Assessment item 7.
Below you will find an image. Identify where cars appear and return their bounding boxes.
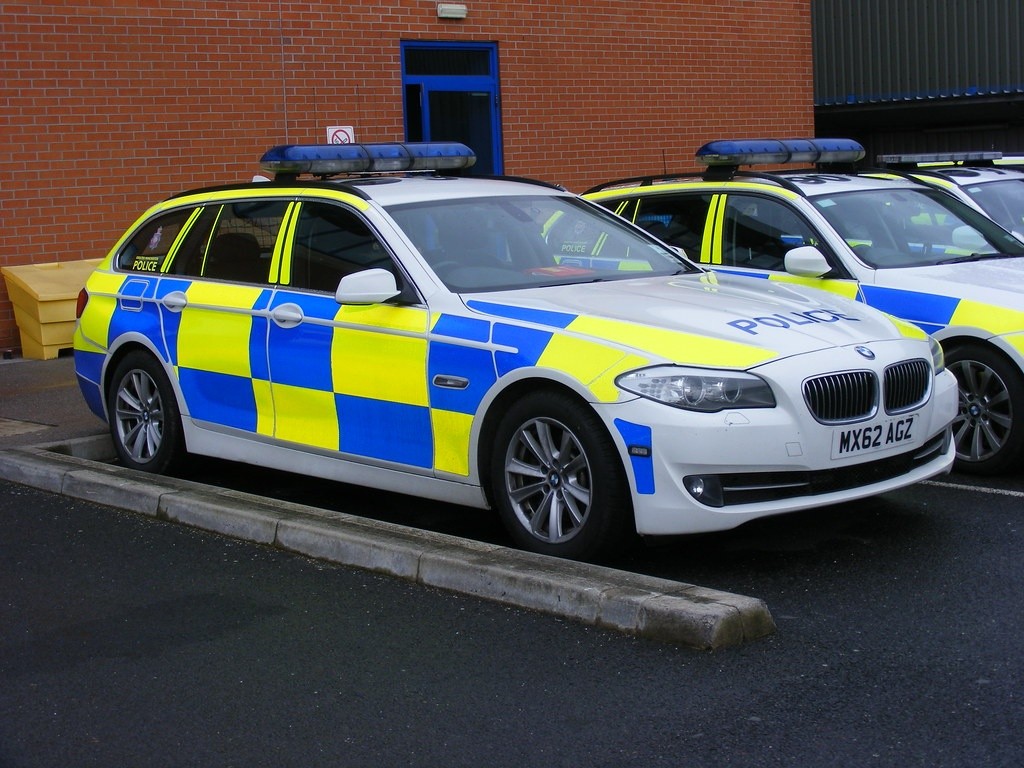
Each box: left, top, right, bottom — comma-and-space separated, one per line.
531, 138, 1024, 479
838, 150, 1024, 260
76, 138, 966, 576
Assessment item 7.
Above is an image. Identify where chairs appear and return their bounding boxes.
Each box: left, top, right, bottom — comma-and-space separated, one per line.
211, 233, 268, 284
